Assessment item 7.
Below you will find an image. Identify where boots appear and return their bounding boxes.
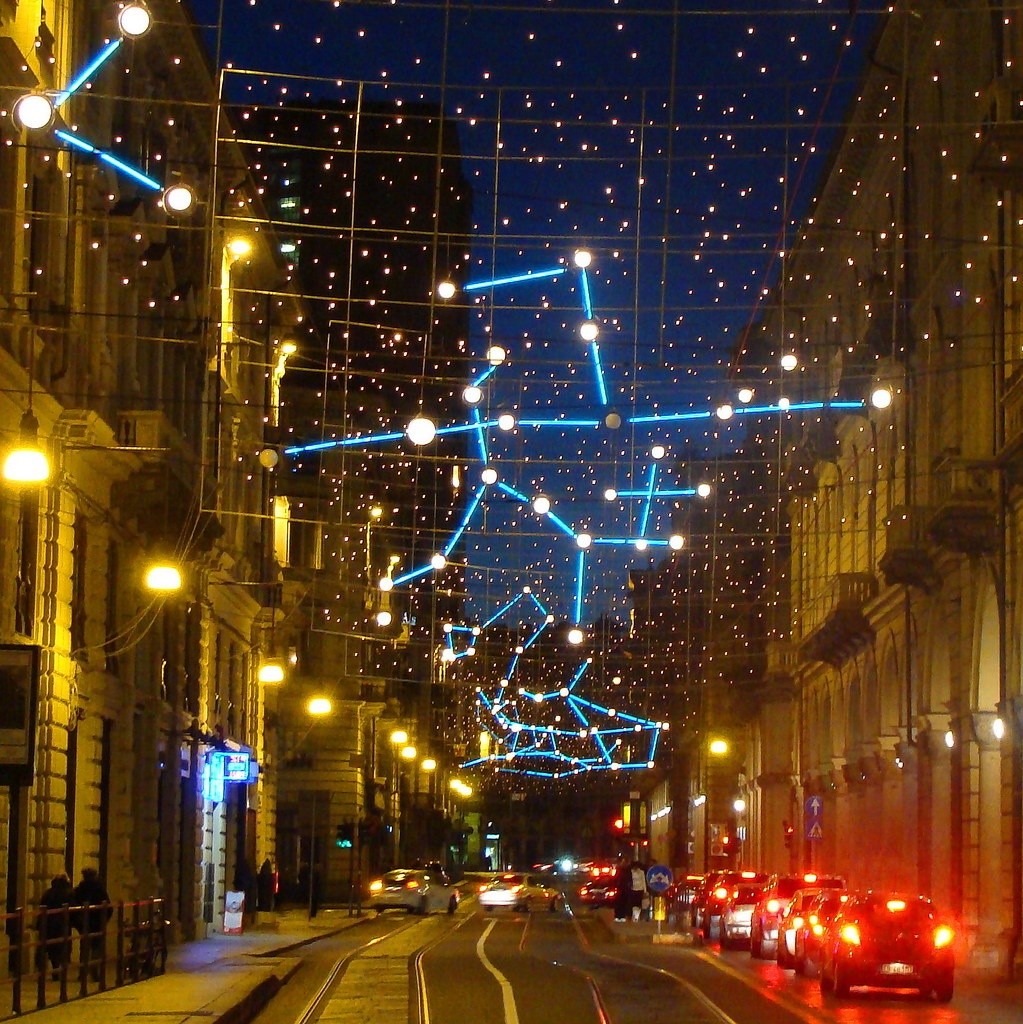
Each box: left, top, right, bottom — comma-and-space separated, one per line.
632, 907, 641, 922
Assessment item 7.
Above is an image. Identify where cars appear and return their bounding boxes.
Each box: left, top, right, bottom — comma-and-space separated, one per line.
369, 868, 460, 915
818, 889, 954, 1004
578, 874, 619, 904
750, 873, 847, 960
795, 890, 852, 978
775, 888, 823, 971
478, 873, 565, 912
669, 868, 768, 938
718, 884, 760, 948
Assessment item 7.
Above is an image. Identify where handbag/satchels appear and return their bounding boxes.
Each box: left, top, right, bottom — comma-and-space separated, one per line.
642, 893, 650, 910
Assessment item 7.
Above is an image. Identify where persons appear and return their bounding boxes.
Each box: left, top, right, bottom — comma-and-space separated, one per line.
631, 862, 647, 923
74, 866, 114, 982
35, 872, 73, 981
613, 860, 634, 922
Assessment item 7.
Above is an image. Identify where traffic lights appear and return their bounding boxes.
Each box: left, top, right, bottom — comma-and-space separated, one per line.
336, 824, 350, 848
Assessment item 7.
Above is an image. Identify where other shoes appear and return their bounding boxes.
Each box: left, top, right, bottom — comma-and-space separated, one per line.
92, 975, 100, 982
52, 973, 59, 980
615, 918, 626, 922
